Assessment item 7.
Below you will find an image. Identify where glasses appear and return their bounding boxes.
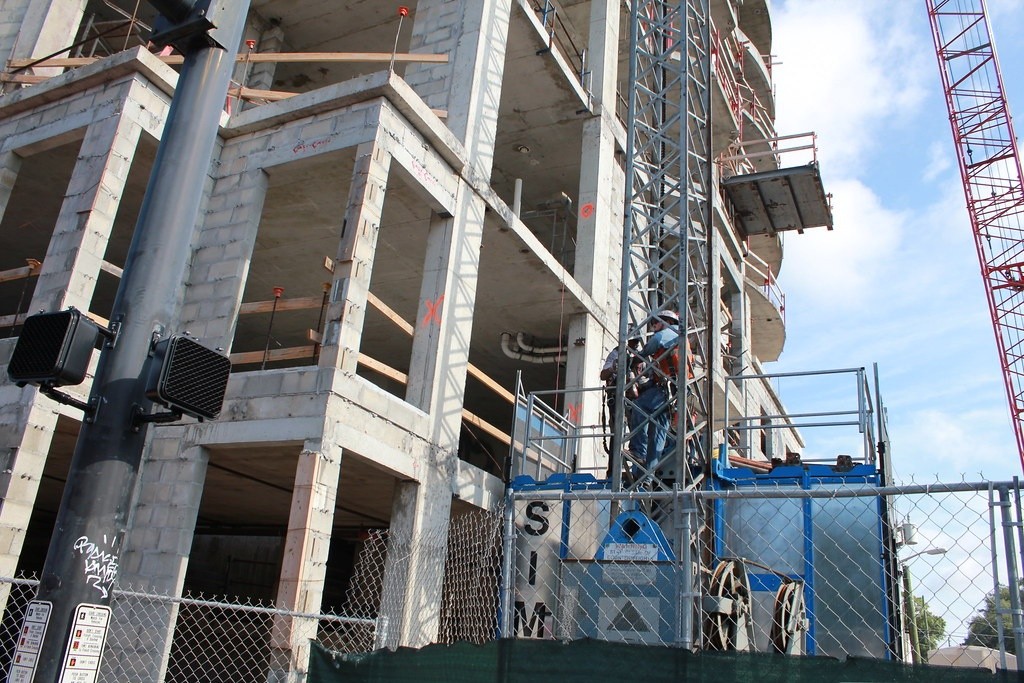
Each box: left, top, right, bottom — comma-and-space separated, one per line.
654, 319, 670, 324
629, 340, 639, 344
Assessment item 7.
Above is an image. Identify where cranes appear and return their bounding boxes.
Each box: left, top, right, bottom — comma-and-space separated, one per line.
503, 1, 1023, 680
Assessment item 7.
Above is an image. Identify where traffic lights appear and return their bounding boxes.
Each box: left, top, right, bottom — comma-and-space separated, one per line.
8, 308, 99, 388
146, 333, 232, 421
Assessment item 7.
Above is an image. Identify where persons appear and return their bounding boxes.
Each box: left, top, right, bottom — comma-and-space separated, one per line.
600, 323, 647, 483
628, 310, 692, 489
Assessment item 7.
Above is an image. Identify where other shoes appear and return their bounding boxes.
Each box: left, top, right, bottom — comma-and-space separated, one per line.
630, 477, 649, 490
624, 450, 645, 464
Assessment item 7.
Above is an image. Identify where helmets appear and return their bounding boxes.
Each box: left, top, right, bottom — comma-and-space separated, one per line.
650, 310, 680, 325
626, 324, 643, 339
